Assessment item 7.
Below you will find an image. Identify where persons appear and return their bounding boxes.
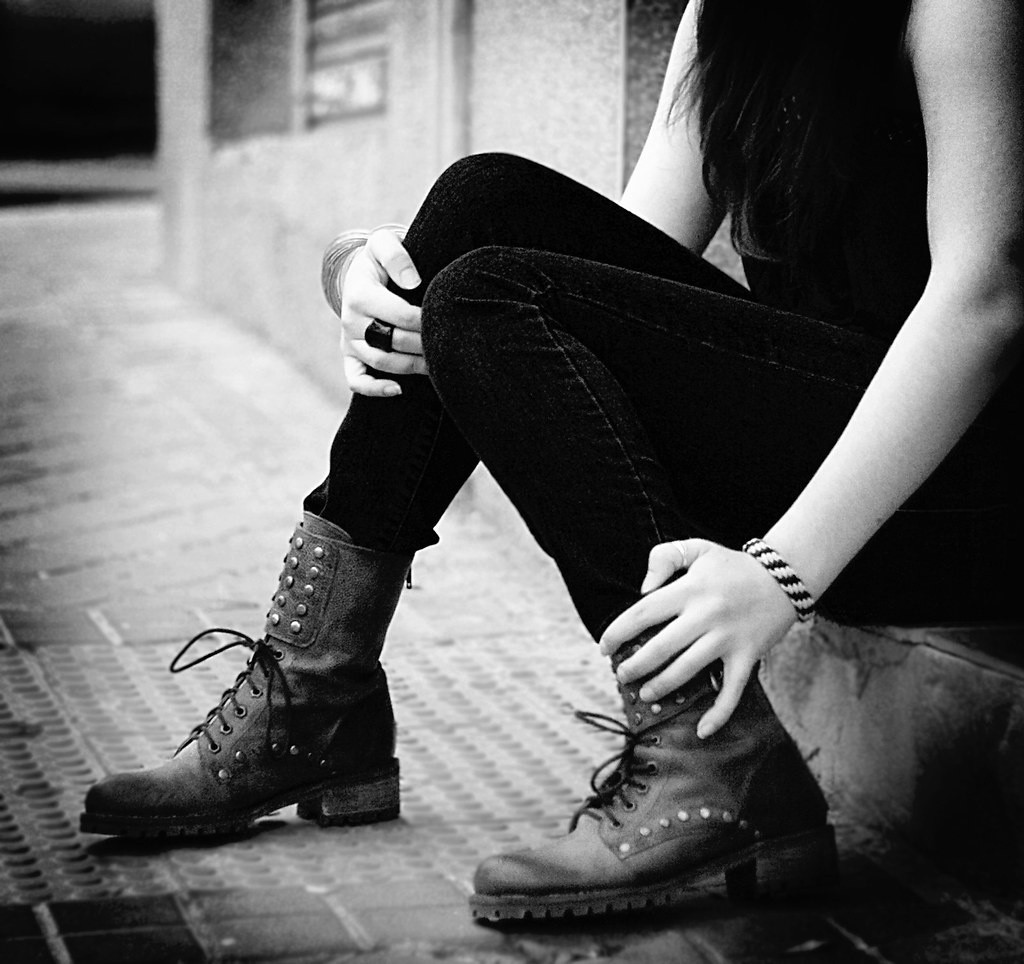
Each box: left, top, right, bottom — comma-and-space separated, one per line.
73, 0, 1023, 928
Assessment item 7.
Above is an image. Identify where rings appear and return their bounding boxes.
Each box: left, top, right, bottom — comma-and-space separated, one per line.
364, 318, 395, 354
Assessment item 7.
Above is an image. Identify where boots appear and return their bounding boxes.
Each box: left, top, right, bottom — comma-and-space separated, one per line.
80, 508, 403, 841
476, 633, 836, 927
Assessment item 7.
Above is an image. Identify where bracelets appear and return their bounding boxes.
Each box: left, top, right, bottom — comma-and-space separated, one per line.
742, 538, 816, 623
